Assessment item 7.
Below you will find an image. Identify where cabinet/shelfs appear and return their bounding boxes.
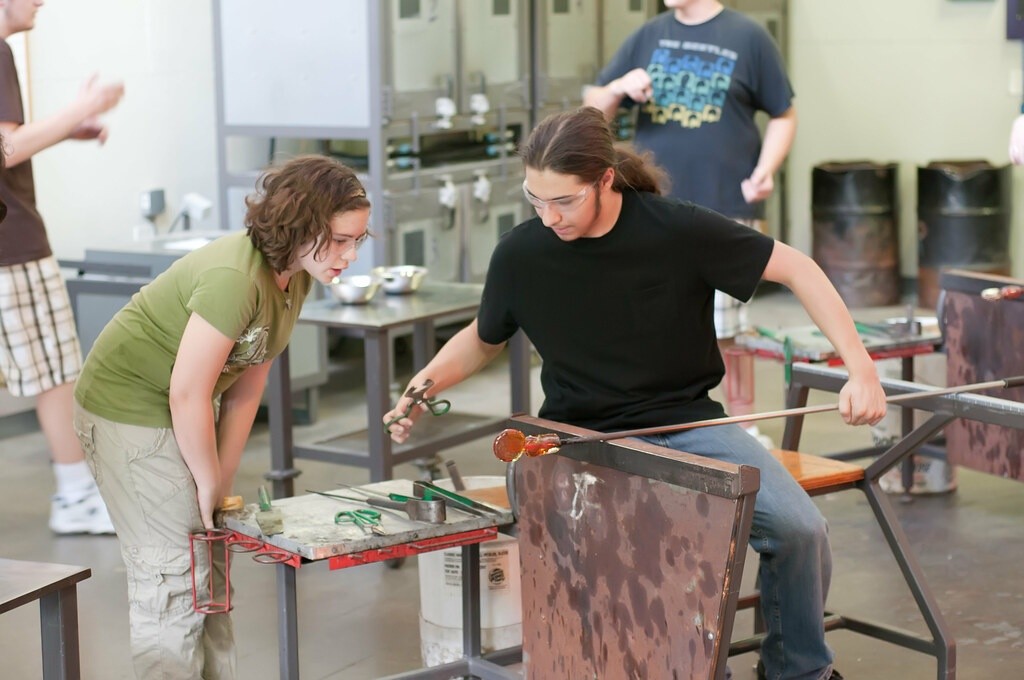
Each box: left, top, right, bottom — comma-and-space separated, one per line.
62, 226, 330, 423
210, 1, 531, 168
532, 1, 661, 128
717, 2, 789, 85
225, 146, 540, 338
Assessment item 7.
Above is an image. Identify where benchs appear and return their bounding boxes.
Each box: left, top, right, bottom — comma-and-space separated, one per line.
211, 360, 1024, 680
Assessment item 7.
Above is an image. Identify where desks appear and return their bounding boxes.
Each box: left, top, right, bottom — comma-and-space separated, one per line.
0, 558, 97, 679
734, 314, 943, 505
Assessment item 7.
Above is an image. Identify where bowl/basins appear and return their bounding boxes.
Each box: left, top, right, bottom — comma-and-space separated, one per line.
376, 264, 425, 293
332, 276, 378, 304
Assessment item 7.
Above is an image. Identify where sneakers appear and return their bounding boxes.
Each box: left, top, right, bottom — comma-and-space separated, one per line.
50, 491, 119, 534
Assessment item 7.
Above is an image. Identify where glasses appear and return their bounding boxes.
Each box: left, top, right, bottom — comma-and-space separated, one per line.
519, 172, 599, 212
319, 235, 368, 255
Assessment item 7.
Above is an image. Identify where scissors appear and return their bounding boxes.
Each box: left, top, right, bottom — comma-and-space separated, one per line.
384, 379, 451, 435
335, 509, 388, 536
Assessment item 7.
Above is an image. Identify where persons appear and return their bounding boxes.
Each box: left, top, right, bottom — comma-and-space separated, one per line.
382, 106, 888, 680
70, 153, 373, 680
0, 0, 124, 536
581, 1, 798, 448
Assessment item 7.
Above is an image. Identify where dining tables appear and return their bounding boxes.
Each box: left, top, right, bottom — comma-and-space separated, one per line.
264, 277, 532, 502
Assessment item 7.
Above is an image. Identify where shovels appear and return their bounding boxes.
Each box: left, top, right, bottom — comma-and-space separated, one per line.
255, 486, 284, 537
